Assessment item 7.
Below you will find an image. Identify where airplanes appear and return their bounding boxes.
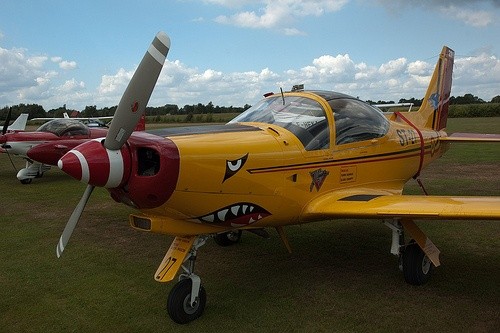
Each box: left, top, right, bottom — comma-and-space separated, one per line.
31, 112, 114, 129
53, 27, 500, 326
264, 90, 414, 134
0, 107, 147, 185
